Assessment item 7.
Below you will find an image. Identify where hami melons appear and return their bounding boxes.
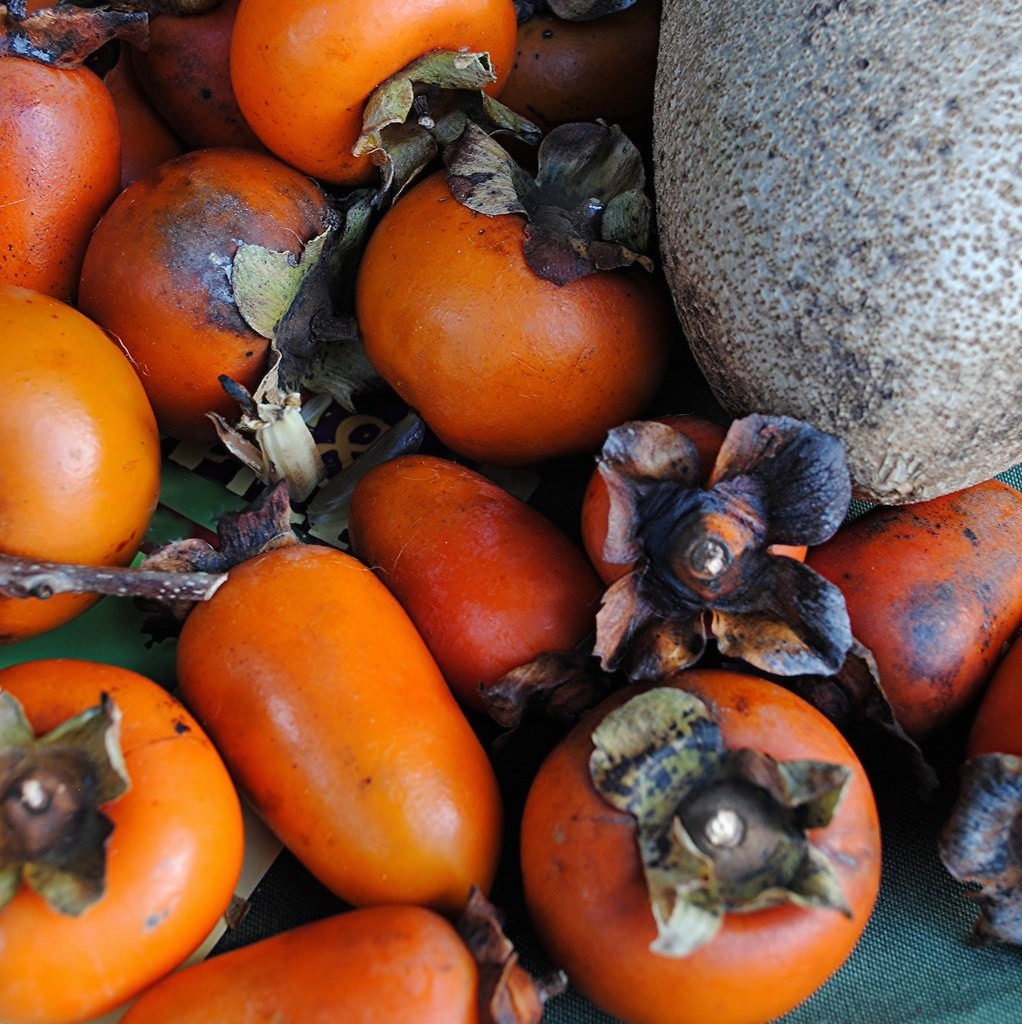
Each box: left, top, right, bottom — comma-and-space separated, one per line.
648, 0, 1022, 517
